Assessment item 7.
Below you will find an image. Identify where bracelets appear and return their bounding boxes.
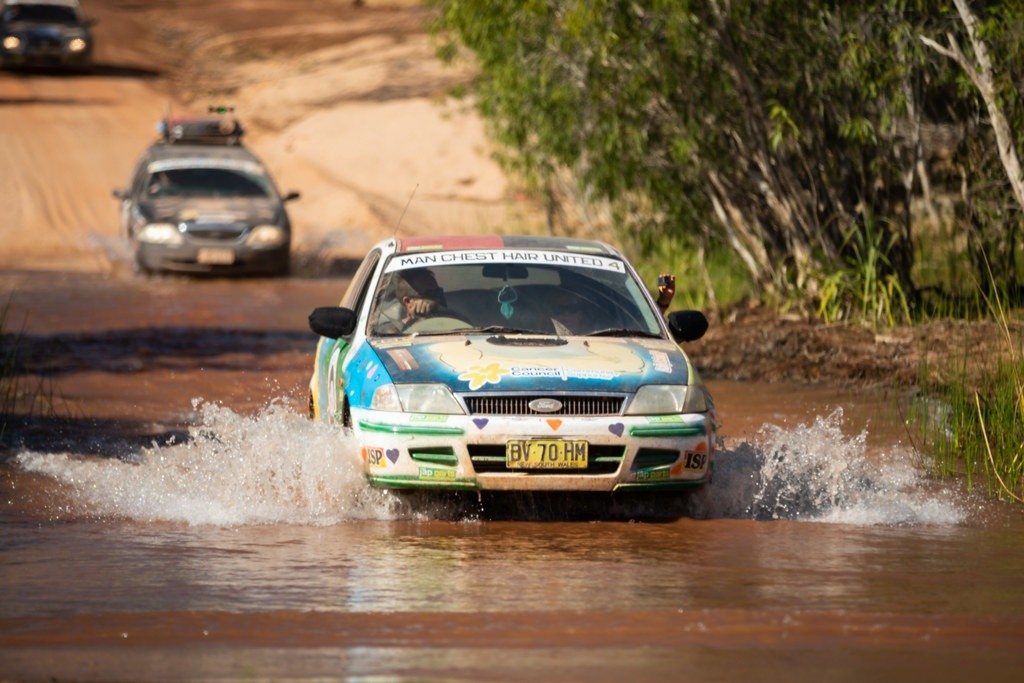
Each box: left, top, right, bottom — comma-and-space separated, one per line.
656, 300, 669, 309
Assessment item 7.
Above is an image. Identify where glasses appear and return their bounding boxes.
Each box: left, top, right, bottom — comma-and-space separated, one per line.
418, 287, 443, 298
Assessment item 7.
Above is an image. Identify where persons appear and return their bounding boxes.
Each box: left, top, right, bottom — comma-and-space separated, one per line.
375, 267, 460, 337
149, 173, 180, 197
539, 273, 675, 337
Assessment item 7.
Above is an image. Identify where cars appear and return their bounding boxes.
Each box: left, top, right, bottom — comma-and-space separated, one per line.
0, 0, 97, 77
110, 116, 301, 281
309, 234, 719, 504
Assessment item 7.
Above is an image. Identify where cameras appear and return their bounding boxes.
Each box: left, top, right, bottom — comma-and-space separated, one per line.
657, 277, 671, 287
